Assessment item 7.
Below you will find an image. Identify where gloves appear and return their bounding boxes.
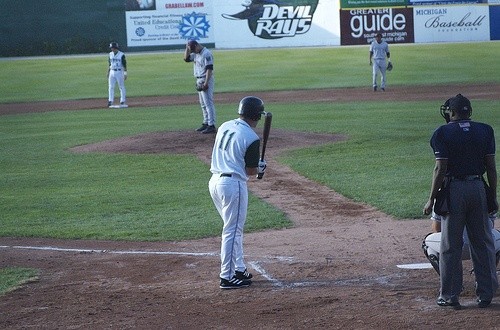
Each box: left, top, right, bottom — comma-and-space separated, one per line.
256, 158, 267, 174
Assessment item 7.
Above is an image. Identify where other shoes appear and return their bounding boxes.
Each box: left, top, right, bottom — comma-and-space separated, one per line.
437, 300, 460, 307
476, 296, 491, 307
195, 124, 216, 134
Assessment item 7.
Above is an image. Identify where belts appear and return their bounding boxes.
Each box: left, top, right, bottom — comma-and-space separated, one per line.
113, 69, 121, 70
452, 175, 480, 181
197, 77, 203, 78
220, 174, 232, 177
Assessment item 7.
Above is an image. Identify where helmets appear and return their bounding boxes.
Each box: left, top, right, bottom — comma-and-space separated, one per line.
109, 42, 119, 49
238, 95, 267, 115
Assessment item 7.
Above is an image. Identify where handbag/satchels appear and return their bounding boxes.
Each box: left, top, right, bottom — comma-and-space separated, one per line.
434, 173, 450, 216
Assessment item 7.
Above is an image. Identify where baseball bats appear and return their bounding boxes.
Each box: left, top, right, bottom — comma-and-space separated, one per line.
255, 112, 272, 180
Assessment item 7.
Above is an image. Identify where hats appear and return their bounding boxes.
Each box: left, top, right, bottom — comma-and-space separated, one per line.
375, 33, 382, 37
188, 41, 198, 53
445, 97, 471, 113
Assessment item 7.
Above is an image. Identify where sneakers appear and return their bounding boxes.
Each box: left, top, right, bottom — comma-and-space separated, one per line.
219, 275, 252, 288
234, 267, 253, 279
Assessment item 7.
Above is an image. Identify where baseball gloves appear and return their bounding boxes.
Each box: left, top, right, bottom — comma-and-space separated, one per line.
196, 80, 209, 92
386, 61, 394, 72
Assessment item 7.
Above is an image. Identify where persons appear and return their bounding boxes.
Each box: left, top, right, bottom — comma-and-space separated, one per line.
183, 40, 217, 134
208, 95, 268, 289
419, 93, 500, 308
369, 34, 391, 93
106, 42, 128, 107
